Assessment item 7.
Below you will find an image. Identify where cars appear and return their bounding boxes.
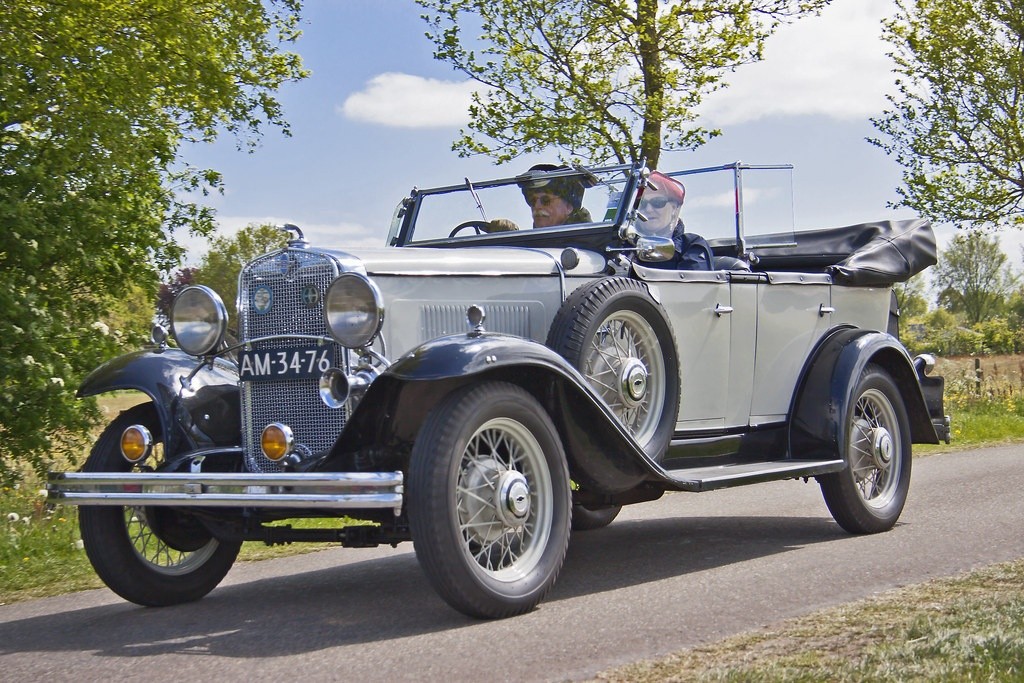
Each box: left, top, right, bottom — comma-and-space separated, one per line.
45, 160, 954, 621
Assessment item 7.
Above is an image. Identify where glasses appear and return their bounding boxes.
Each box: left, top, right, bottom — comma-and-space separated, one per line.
639, 196, 675, 209
530, 194, 560, 207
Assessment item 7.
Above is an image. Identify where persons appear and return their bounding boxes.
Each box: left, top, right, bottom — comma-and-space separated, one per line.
485, 164, 592, 233
634, 170, 715, 270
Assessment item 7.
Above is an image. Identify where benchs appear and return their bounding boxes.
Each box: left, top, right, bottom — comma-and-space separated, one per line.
713, 256, 752, 272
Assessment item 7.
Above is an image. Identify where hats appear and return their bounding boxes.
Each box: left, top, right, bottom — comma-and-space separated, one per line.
519, 164, 585, 210
643, 171, 685, 205
